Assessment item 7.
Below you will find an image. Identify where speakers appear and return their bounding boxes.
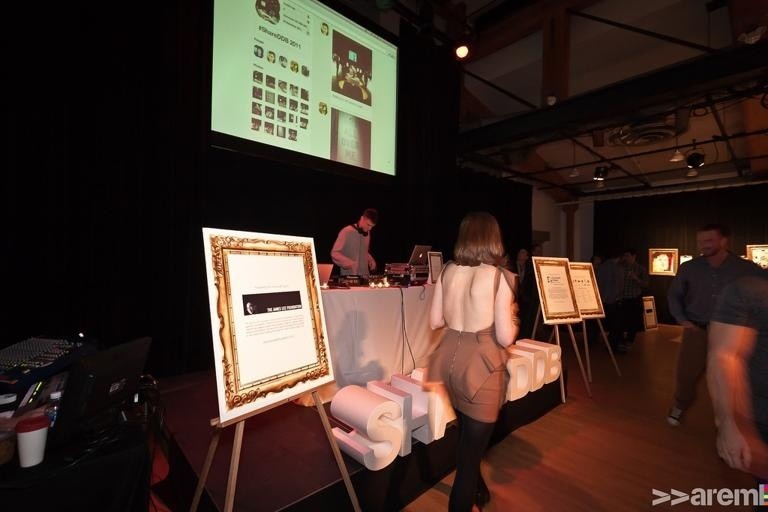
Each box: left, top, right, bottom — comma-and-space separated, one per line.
0, 423, 153, 511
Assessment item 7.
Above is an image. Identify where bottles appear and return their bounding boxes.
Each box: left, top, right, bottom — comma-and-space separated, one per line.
45, 392, 62, 427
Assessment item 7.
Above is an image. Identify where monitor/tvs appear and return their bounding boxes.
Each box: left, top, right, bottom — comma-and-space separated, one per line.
203, 0, 407, 190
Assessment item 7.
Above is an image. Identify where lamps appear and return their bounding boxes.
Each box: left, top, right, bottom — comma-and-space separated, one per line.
593, 165, 620, 182
669, 135, 685, 162
684, 138, 706, 169
568, 142, 579, 177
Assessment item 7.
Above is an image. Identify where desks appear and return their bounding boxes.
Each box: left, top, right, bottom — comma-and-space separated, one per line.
292, 283, 435, 407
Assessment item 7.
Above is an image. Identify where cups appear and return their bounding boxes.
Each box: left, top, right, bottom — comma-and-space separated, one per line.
15, 416, 51, 467
0, 393, 17, 419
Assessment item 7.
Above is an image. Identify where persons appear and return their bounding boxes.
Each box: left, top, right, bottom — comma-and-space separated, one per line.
425, 211, 519, 511
331, 206, 379, 276
704, 268, 768, 512
587, 248, 651, 354
512, 242, 547, 342
666, 220, 760, 428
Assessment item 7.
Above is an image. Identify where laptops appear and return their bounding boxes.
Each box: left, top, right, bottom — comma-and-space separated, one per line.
392, 245, 432, 265
317, 263, 334, 285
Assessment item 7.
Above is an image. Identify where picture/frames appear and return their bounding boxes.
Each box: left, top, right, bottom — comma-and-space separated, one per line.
648, 248, 678, 276
746, 245, 768, 269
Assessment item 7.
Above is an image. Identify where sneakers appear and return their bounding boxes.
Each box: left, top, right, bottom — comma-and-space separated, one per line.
666, 405, 682, 426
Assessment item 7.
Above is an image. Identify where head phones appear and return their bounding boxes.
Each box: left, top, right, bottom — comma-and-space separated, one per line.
356, 222, 368, 237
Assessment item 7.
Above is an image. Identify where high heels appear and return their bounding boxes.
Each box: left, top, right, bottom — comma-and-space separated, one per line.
474, 491, 489, 511
